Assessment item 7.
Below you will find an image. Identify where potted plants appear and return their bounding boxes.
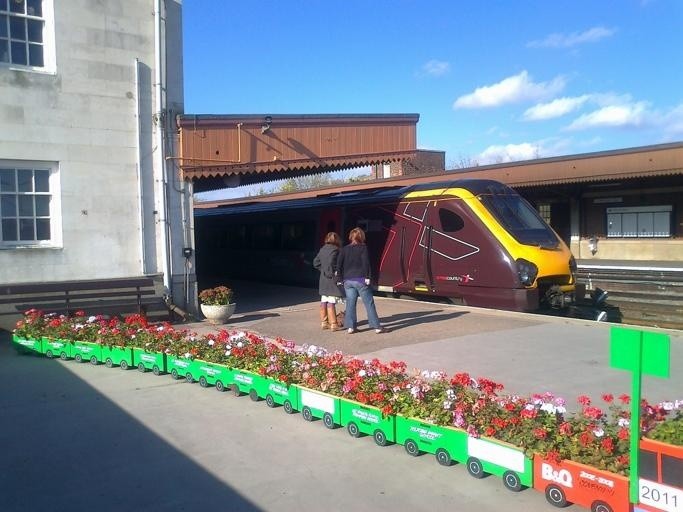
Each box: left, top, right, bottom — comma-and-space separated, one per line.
199, 286, 236, 326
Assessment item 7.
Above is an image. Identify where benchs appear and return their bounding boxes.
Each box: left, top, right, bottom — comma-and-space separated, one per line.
7, 279, 174, 323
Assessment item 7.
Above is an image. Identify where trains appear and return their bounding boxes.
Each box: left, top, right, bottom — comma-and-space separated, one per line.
191, 178, 577, 314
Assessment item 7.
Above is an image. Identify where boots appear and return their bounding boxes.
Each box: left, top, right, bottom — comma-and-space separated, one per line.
318, 305, 330, 330
325, 306, 341, 332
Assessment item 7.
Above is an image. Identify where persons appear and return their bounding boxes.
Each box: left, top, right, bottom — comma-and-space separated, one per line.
312, 232, 344, 332
334, 226, 384, 334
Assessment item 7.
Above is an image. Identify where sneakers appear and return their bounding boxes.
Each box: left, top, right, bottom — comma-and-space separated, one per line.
347, 326, 392, 334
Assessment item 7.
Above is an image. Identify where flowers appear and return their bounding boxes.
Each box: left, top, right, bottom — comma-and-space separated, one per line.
265, 337, 408, 417
407, 368, 682, 475
13, 307, 125, 346
124, 314, 267, 377
